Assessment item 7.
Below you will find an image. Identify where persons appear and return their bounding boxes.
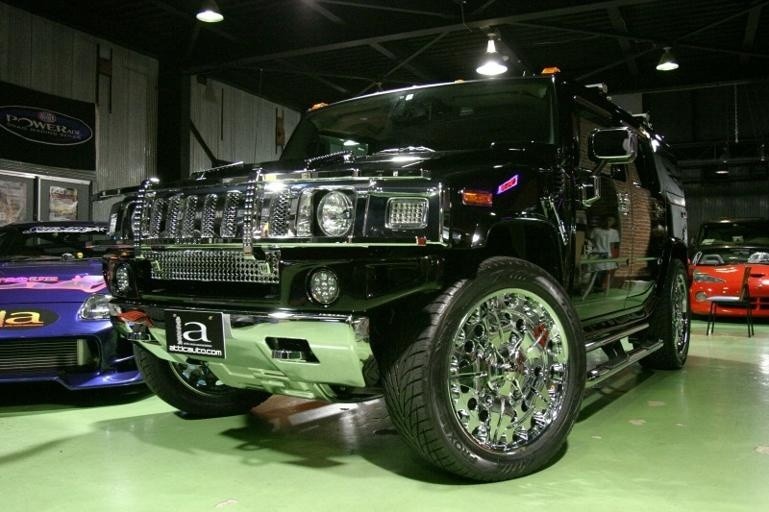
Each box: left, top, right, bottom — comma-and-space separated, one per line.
588, 214, 621, 294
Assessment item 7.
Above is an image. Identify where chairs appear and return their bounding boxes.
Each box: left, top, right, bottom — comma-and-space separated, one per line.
699, 250, 769, 266
705, 265, 754, 337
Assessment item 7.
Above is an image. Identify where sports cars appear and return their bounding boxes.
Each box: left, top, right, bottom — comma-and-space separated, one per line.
0, 218, 152, 402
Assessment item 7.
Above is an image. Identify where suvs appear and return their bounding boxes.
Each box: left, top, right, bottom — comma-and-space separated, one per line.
93, 62, 700, 487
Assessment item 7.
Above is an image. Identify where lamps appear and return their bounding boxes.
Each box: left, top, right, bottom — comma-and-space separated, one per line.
473, 25, 509, 77
194, 0, 224, 23
655, 43, 680, 72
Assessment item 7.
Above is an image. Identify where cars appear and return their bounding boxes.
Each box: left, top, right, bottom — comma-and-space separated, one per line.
685, 216, 769, 323
